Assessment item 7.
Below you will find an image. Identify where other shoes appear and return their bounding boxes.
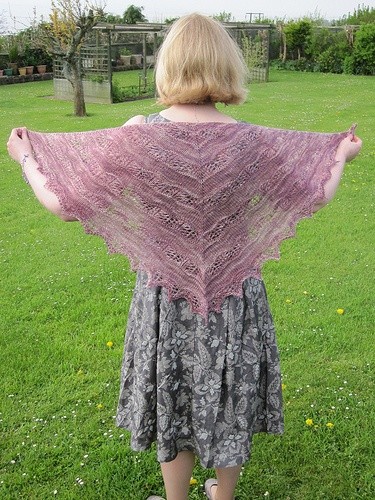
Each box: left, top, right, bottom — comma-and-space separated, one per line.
145, 495, 168, 500
205, 478, 235, 500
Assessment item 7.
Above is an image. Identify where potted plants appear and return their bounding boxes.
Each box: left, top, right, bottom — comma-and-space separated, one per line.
0, 62, 5, 76
4, 62, 13, 75
18, 59, 27, 76
110, 52, 117, 66
7, 43, 19, 75
131, 42, 142, 64
119, 47, 132, 66
36, 48, 48, 74
23, 48, 35, 75
146, 46, 155, 64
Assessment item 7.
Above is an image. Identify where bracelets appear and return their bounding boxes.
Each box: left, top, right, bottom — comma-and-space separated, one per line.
21, 153, 35, 185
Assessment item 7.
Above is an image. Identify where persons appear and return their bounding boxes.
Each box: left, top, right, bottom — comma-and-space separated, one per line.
6, 12, 362, 499
